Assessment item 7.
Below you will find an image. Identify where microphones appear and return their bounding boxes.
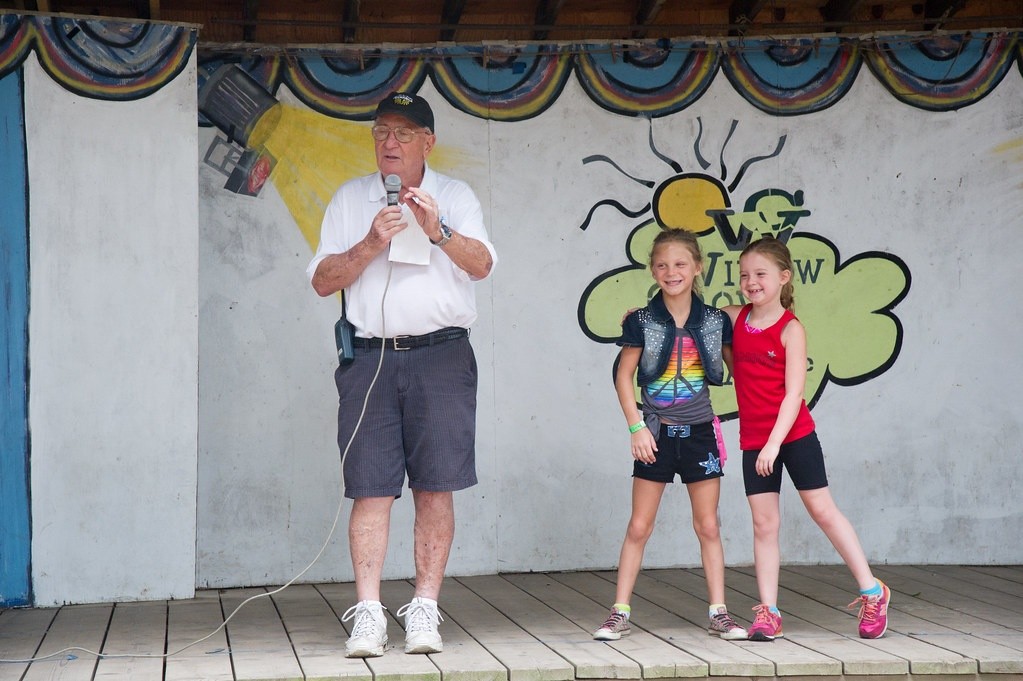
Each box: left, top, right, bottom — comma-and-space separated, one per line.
385, 174, 402, 207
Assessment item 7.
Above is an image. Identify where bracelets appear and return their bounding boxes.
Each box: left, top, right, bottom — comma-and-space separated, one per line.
629, 421, 646, 433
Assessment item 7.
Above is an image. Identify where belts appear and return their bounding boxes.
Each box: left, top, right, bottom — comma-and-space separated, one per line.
352, 328, 468, 350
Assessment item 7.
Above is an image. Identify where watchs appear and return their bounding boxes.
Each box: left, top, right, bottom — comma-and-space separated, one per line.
429, 224, 451, 247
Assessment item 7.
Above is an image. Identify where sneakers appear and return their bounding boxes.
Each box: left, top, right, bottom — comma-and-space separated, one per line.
708, 606, 748, 640
593, 607, 632, 640
341, 600, 390, 658
748, 604, 785, 641
848, 577, 891, 638
396, 597, 444, 653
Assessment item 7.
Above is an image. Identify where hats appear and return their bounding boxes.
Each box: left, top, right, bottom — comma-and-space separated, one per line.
374, 91, 434, 134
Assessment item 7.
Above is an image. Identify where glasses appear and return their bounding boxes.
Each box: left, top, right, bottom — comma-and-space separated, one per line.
372, 124, 428, 143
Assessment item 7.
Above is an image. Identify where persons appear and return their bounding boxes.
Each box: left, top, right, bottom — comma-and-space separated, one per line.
621, 237, 890, 641
592, 229, 747, 640
307, 91, 498, 658
